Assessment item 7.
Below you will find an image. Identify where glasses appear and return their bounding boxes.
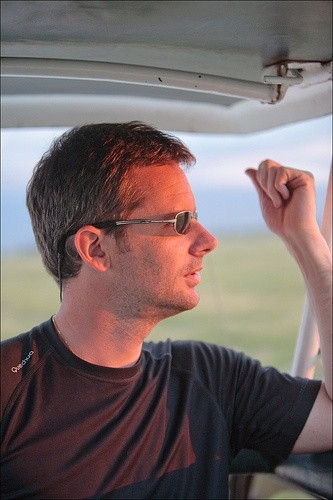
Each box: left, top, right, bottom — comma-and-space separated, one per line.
57, 207, 197, 253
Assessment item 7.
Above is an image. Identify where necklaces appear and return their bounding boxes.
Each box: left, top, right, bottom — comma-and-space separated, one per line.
52, 315, 75, 355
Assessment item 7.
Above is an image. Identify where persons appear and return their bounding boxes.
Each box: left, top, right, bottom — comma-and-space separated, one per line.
1, 120, 332, 499
